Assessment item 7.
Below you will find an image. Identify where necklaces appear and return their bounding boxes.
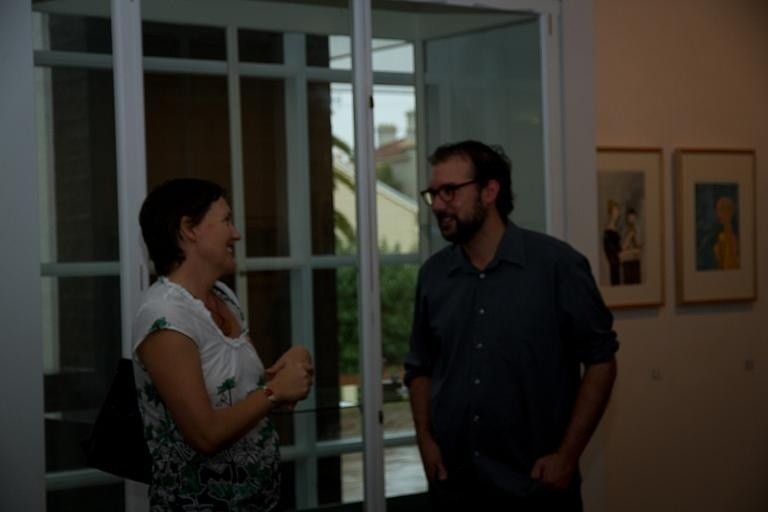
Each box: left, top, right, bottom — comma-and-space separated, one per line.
195, 294, 232, 336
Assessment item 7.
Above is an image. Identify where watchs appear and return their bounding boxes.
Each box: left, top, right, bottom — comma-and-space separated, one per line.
262, 386, 278, 409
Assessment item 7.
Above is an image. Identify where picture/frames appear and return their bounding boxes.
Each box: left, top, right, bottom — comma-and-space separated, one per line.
592, 145, 665, 310
674, 148, 758, 306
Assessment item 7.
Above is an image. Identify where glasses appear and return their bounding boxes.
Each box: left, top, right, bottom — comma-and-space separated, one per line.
421, 179, 484, 201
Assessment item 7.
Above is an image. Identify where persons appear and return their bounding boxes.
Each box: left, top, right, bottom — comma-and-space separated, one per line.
602, 199, 624, 286
132, 179, 314, 510
405, 141, 621, 510
621, 210, 641, 286
712, 195, 739, 268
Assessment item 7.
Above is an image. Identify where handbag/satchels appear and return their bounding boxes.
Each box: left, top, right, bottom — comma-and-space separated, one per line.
83, 358, 153, 486
